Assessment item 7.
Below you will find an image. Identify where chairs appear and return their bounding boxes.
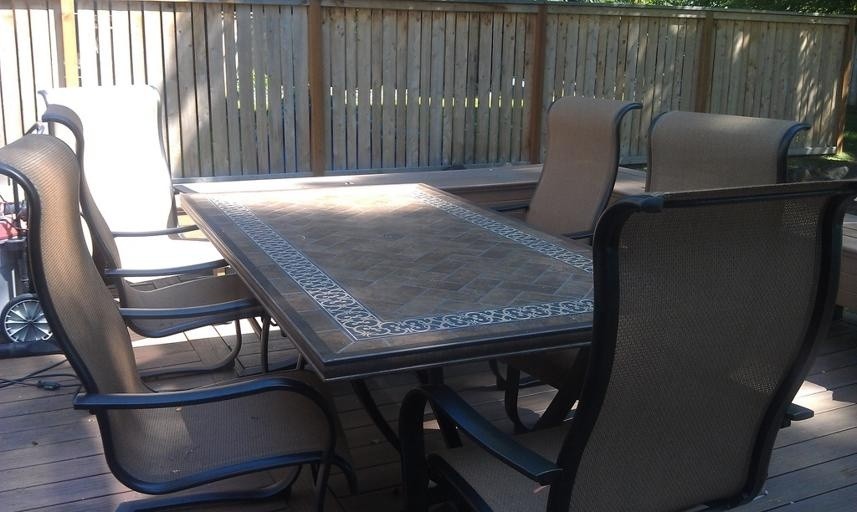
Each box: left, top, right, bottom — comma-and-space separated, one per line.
1, 132, 366, 512
41, 103, 306, 380
398, 177, 857, 511
433, 92, 644, 390
505, 109, 812, 436
39, 84, 234, 290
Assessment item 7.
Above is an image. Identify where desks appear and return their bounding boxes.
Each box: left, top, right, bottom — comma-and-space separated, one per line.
174, 174, 596, 512
